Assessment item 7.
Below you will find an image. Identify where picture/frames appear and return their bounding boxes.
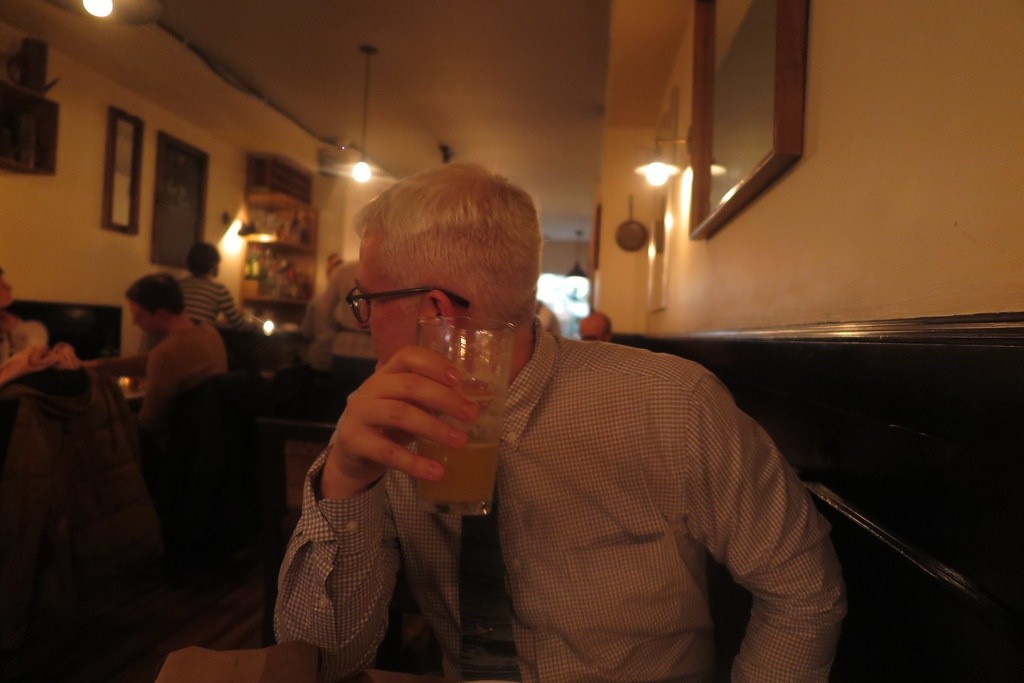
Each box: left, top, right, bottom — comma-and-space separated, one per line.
100, 106, 144, 236
689, 0, 807, 242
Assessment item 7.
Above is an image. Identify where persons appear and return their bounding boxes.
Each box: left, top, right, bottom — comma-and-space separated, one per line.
271, 160, 845, 683
123, 271, 230, 435
0, 270, 50, 400
178, 242, 253, 333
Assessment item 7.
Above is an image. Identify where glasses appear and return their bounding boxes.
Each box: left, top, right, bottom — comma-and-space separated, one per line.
346, 286, 471, 323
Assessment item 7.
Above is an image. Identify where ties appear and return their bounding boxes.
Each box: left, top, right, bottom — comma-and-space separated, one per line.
458, 480, 522, 682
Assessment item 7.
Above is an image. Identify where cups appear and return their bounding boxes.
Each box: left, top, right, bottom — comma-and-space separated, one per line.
416, 315, 516, 515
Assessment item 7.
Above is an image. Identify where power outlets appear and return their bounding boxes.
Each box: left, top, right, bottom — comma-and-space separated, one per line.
238, 189, 318, 306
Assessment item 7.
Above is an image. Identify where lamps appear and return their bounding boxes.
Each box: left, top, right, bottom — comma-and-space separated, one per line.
222, 211, 275, 242
353, 46, 377, 182
566, 232, 589, 282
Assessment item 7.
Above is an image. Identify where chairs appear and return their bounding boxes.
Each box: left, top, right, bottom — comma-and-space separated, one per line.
0, 359, 323, 653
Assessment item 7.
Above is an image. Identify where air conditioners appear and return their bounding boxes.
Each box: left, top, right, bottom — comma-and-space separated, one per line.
635, 137, 686, 186
